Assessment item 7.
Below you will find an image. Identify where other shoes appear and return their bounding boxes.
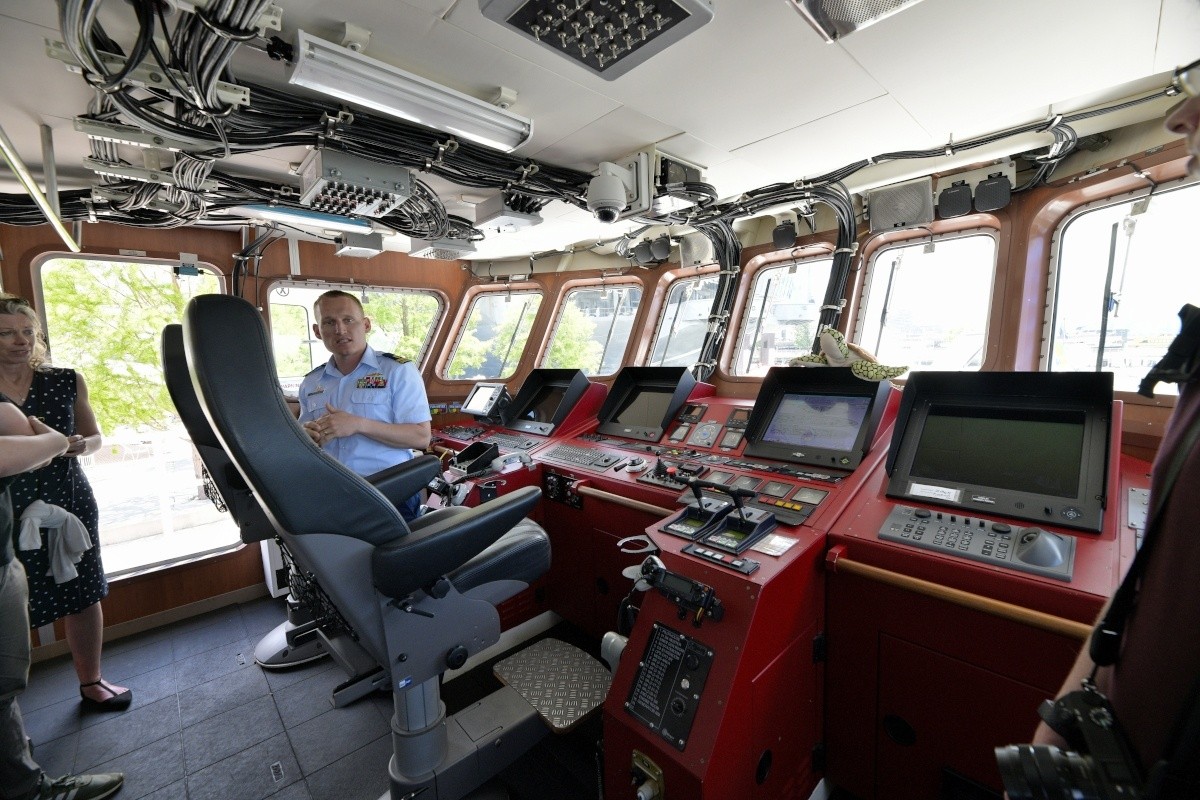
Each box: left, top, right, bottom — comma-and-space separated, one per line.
79, 676, 134, 708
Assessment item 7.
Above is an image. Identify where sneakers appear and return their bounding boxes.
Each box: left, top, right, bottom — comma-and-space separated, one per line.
32, 771, 125, 800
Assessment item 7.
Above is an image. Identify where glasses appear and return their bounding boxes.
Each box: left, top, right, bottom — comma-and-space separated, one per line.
1175, 59, 1200, 98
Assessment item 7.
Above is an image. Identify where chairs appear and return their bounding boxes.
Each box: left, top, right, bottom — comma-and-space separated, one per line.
161, 295, 559, 800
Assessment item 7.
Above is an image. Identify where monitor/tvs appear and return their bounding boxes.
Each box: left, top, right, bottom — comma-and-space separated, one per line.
761, 392, 872, 451
909, 404, 1085, 500
610, 385, 675, 429
460, 382, 506, 416
519, 386, 568, 424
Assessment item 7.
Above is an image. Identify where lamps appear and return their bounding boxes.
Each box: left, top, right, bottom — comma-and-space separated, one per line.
281, 31, 534, 153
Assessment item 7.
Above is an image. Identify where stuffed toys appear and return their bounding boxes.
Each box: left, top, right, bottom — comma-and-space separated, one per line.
789, 328, 911, 382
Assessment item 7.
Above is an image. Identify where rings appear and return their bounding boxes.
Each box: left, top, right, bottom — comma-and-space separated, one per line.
323, 430, 327, 436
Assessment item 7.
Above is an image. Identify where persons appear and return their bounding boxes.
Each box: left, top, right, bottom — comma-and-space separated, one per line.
0, 293, 132, 710
0, 402, 124, 800
296, 290, 432, 523
1003, 303, 1200, 800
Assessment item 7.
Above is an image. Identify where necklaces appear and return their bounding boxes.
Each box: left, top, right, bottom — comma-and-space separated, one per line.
0, 371, 30, 402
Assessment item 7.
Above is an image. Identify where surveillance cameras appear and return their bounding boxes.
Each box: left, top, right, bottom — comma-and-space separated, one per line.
587, 176, 627, 224
